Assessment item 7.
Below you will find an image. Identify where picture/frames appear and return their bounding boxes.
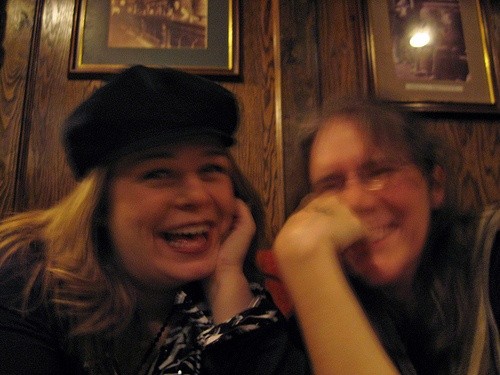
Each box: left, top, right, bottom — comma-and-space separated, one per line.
67, 0, 244, 82
360, 0, 500, 117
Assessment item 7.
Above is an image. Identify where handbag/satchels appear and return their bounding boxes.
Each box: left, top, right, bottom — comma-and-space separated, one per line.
458, 205, 500, 375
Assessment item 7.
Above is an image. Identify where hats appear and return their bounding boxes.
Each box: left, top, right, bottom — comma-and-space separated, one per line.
62, 65, 241, 181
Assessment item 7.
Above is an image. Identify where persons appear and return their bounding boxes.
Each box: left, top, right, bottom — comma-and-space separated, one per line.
272, 101, 500, 375
131, 1, 189, 47
0, 64, 306, 375
394, 0, 460, 78
